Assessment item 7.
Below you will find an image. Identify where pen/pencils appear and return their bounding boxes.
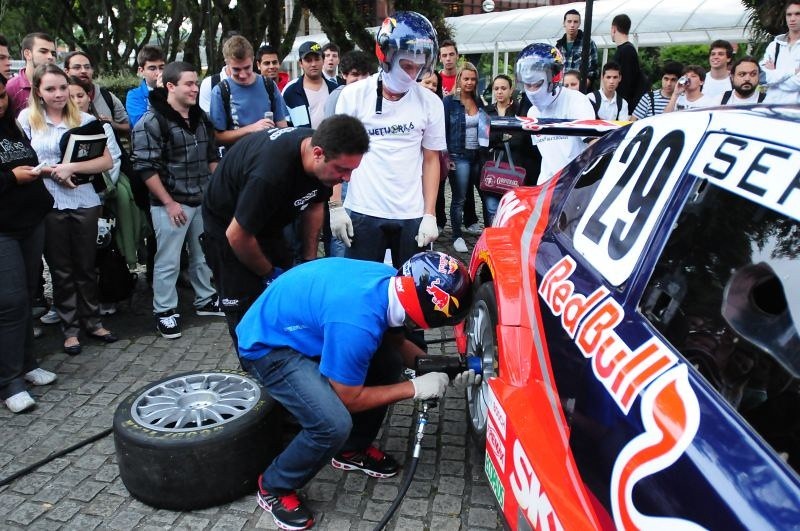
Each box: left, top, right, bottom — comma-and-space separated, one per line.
32, 161, 47, 171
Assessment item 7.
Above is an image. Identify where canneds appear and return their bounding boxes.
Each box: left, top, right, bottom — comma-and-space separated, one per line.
679, 77, 690, 85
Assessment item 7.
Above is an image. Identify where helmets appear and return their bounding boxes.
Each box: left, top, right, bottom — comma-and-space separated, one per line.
395, 248, 472, 330
516, 42, 565, 93
375, 10, 439, 73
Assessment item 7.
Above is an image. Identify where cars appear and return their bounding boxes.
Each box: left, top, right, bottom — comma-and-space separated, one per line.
457, 103, 799, 530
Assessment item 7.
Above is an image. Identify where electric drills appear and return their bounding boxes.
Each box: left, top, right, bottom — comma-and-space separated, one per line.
416, 354, 481, 406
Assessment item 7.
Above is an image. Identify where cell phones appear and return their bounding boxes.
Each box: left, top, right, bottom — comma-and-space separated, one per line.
264, 111, 274, 121
33, 159, 48, 171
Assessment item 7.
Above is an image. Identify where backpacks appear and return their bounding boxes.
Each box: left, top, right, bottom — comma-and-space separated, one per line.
96, 231, 137, 306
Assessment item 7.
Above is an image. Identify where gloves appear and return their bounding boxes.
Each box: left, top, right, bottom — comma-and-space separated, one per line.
415, 215, 439, 250
453, 369, 483, 390
410, 371, 450, 402
330, 206, 354, 248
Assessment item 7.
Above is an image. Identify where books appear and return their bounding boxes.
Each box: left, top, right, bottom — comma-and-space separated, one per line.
63, 134, 108, 185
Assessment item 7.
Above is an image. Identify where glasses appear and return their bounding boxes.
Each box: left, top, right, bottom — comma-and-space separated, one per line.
68, 65, 92, 71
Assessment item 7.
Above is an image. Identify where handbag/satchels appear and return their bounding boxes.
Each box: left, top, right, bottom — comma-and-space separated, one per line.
478, 139, 527, 195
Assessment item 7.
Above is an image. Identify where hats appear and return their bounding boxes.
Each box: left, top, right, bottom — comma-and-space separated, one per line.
300, 41, 322, 59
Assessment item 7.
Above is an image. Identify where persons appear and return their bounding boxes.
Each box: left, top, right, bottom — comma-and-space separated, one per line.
701, 40, 734, 101
199, 114, 369, 430
235, 250, 481, 531
586, 63, 628, 121
713, 57, 772, 105
556, 9, 599, 94
611, 14, 640, 107
665, 313, 800, 474
664, 65, 712, 112
564, 70, 582, 91
759, 0, 800, 103
329, 11, 446, 381
0, 32, 226, 412
419, 41, 542, 252
630, 64, 682, 121
515, 43, 598, 186
198, 31, 372, 264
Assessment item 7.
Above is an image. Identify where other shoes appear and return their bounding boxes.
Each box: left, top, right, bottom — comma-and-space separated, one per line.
40, 310, 62, 323
32, 306, 46, 317
98, 300, 116, 316
87, 326, 117, 342
467, 224, 485, 235
63, 338, 82, 354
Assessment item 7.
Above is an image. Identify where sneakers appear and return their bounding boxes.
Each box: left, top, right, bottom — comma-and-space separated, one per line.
331, 446, 399, 479
155, 308, 183, 340
256, 474, 315, 530
195, 295, 227, 317
5, 391, 36, 413
24, 366, 57, 386
454, 238, 468, 252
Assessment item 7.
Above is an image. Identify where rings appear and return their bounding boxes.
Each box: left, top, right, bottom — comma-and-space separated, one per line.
176, 218, 178, 220
